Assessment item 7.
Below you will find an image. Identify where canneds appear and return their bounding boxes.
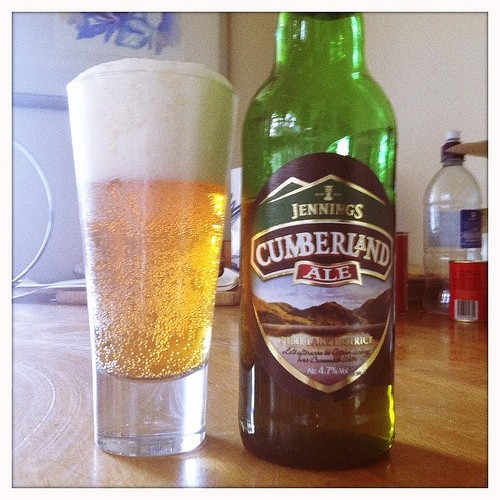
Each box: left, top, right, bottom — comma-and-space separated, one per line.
448, 259, 487, 323
394, 231, 410, 315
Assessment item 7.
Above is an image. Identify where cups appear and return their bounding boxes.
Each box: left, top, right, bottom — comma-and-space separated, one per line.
67, 71, 240, 457
448, 260, 488, 323
395, 232, 408, 320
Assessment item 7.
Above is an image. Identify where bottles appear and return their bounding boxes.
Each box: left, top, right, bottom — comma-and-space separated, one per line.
237, 12, 398, 471
423, 129, 483, 315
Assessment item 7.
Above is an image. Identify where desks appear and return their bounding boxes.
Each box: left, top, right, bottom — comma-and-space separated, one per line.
13, 280, 488, 487
